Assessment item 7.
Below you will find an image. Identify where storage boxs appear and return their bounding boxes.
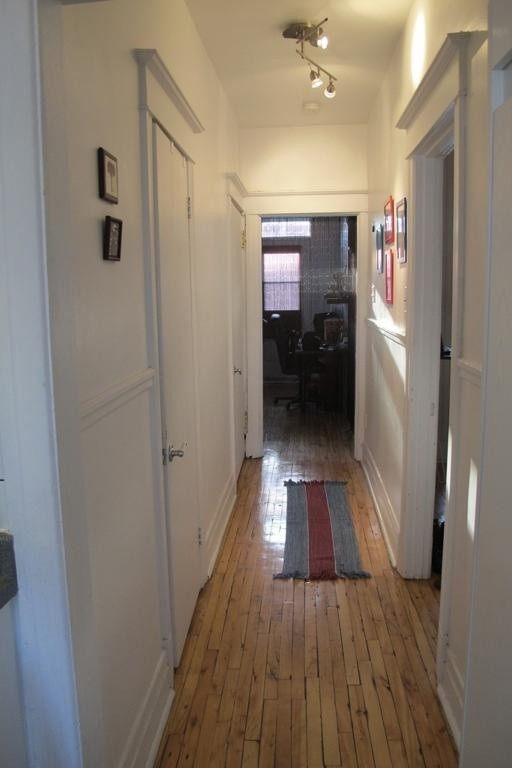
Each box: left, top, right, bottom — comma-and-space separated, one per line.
320, 317, 344, 346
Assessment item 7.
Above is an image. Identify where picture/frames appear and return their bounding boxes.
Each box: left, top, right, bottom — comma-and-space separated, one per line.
372, 193, 410, 311
102, 214, 127, 264
95, 143, 123, 206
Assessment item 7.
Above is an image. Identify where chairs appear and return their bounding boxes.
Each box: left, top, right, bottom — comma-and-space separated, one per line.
271, 308, 323, 414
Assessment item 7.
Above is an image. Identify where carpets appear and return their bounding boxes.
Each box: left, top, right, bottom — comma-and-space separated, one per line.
272, 476, 374, 585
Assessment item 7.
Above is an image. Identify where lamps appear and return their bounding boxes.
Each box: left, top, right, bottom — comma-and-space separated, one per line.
281, 14, 346, 102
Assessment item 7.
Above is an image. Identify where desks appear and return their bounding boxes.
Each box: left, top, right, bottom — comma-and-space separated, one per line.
297, 347, 353, 418
